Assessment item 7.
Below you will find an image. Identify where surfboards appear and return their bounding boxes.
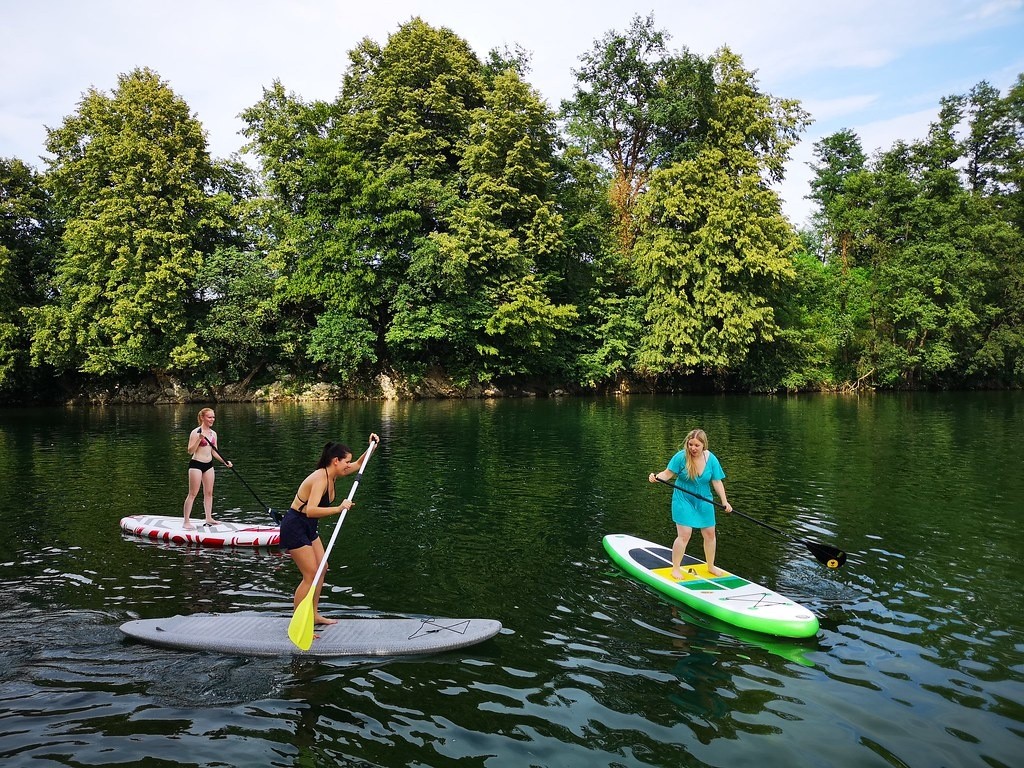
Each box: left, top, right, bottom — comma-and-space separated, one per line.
601, 531, 821, 639
119, 514, 280, 547
118, 616, 503, 653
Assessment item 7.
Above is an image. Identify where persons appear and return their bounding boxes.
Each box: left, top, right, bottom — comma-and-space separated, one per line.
183, 408, 233, 530
649, 429, 733, 580
280, 433, 380, 640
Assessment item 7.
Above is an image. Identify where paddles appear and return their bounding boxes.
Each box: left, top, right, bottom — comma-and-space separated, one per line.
286, 435, 380, 654
197, 427, 285, 529
652, 473, 849, 570
